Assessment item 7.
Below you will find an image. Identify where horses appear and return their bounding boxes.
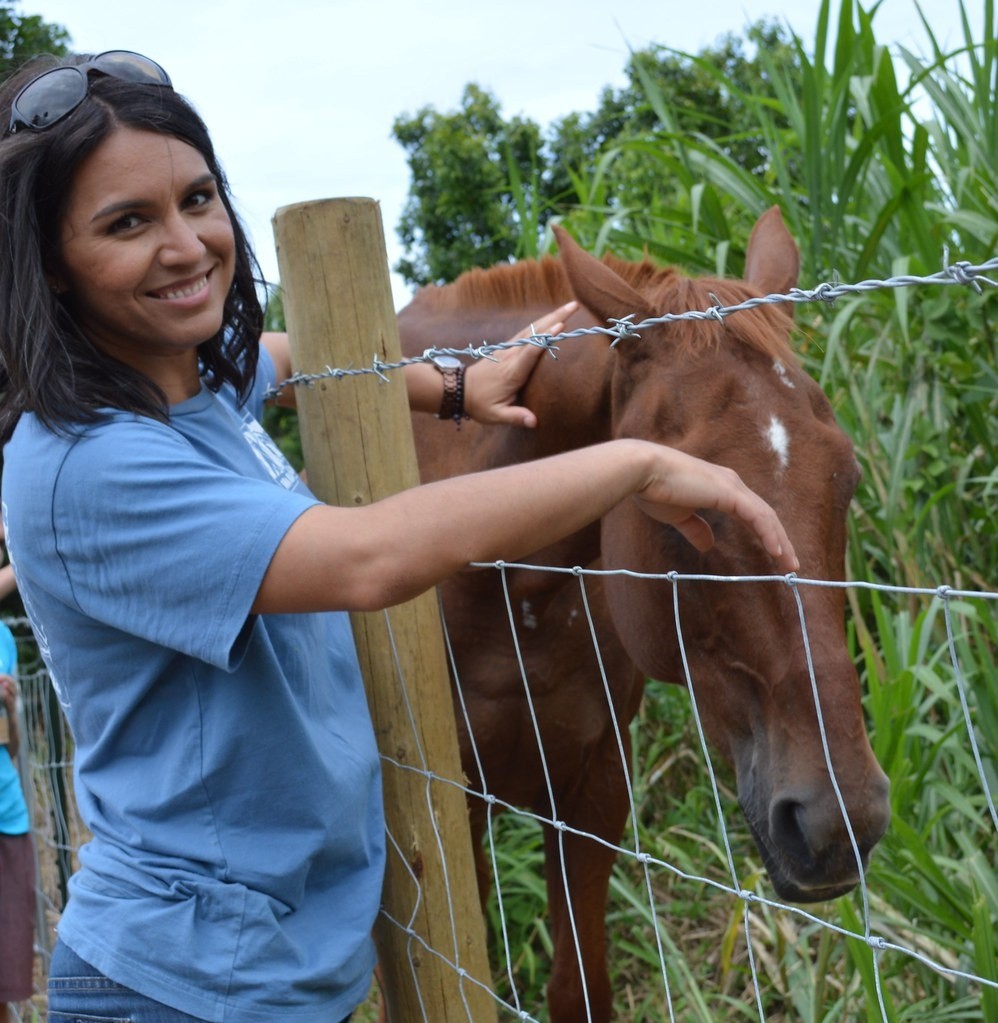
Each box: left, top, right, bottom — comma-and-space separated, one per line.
393, 203, 892, 1021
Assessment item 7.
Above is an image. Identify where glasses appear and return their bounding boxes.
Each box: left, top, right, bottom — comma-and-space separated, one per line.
9, 49, 173, 131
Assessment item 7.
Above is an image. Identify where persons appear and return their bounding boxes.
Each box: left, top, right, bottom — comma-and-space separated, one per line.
0, 54, 804, 1022
0, 563, 40, 1020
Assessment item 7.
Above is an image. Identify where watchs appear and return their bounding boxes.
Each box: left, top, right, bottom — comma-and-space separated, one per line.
433, 355, 462, 420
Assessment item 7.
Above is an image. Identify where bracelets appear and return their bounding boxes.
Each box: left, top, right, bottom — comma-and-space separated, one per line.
455, 361, 466, 432
